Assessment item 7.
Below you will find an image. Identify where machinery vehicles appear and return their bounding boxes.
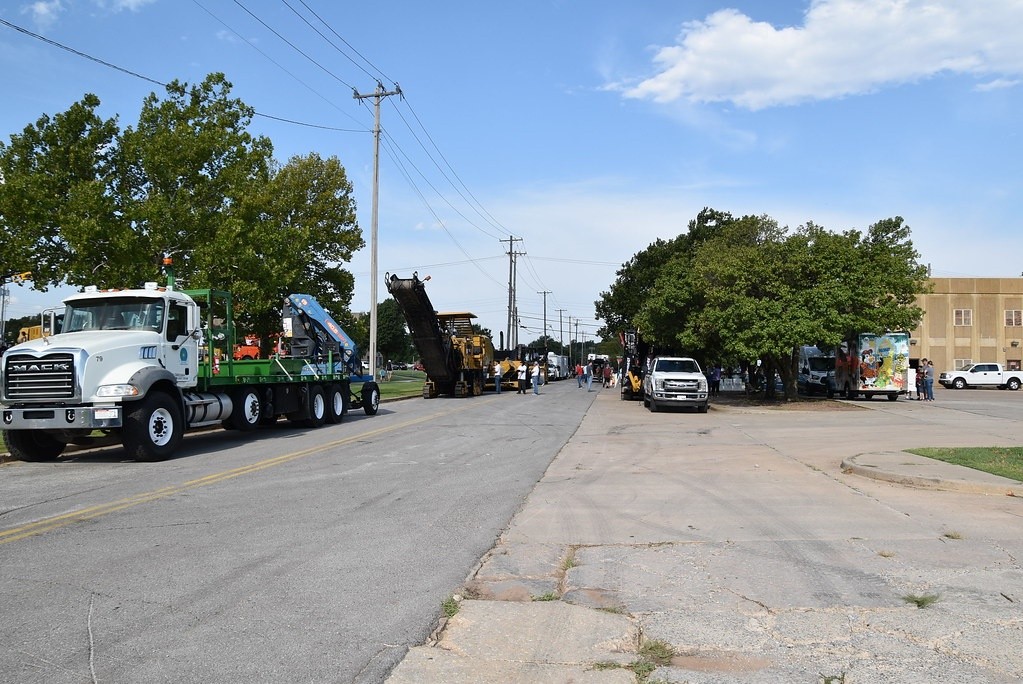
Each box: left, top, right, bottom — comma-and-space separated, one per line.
384, 271, 548, 399
232, 331, 290, 361
619, 327, 648, 400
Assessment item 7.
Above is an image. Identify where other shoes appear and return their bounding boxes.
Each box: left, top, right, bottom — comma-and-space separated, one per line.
523, 392, 526, 394
532, 393, 538, 395
517, 392, 521, 394
606, 386, 609, 388
603, 384, 604, 387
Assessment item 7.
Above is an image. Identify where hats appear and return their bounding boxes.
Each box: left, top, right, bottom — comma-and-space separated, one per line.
534, 361, 538, 365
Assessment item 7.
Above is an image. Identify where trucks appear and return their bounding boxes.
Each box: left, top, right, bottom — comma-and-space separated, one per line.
547, 351, 569, 380
798, 346, 836, 396
826, 331, 909, 401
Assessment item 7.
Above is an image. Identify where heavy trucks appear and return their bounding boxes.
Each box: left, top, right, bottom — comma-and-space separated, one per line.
0, 257, 380, 461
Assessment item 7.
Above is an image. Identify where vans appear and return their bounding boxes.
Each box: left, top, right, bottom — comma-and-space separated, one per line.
587, 353, 609, 383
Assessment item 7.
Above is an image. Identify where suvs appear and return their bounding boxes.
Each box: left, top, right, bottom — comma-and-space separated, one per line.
642, 356, 708, 413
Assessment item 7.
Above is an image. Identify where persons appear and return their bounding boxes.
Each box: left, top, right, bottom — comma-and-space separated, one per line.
706, 364, 721, 398
379, 360, 393, 382
915, 358, 935, 401
20, 331, 27, 343
516, 360, 527, 394
6, 331, 15, 348
575, 361, 594, 392
531, 361, 539, 396
602, 363, 611, 389
596, 366, 602, 383
905, 364, 914, 399
492, 360, 502, 394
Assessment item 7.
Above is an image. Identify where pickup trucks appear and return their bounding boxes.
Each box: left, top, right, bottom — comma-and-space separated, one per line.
938, 362, 1023, 391
